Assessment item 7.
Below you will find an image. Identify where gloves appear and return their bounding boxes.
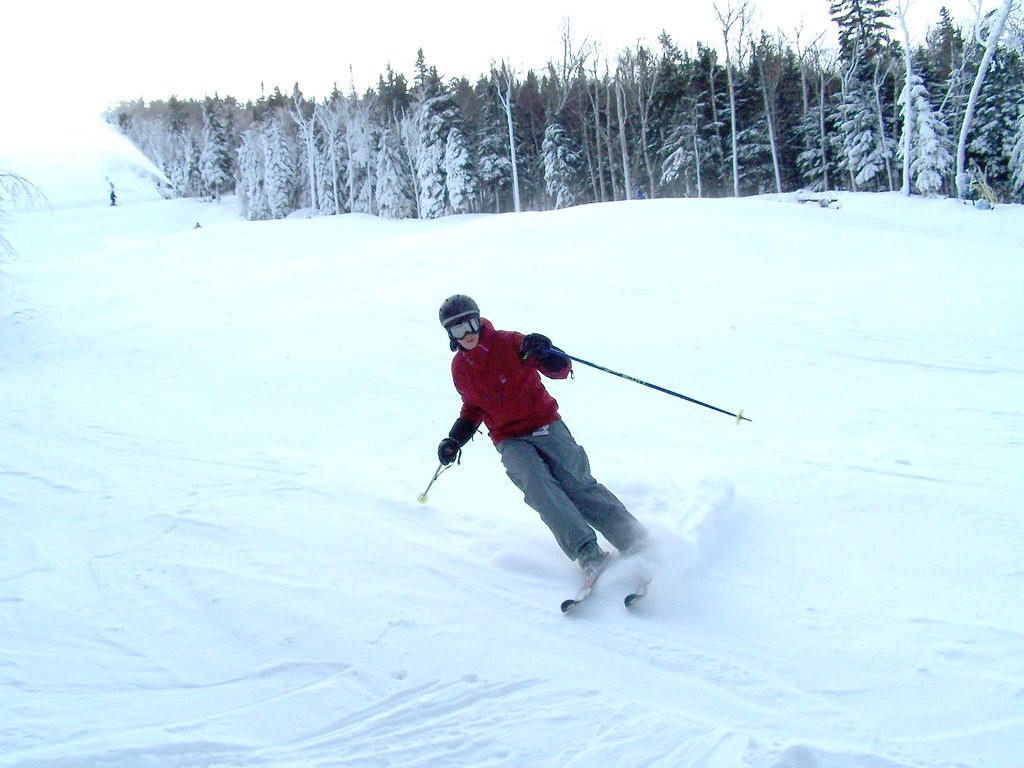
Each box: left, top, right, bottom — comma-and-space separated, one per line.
438, 418, 478, 466
521, 333, 566, 372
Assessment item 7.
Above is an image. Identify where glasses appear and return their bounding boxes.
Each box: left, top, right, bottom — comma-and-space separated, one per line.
448, 316, 481, 339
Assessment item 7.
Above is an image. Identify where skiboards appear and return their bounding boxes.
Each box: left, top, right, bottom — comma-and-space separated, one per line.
561, 550, 652, 615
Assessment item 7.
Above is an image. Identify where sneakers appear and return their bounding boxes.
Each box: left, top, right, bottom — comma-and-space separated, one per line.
577, 542, 602, 569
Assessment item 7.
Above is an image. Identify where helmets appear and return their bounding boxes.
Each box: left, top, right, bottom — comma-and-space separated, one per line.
439, 295, 480, 328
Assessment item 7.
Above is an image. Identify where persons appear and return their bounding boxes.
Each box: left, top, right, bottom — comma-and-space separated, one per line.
434, 294, 669, 611
193, 222, 202, 229
109, 191, 117, 206
